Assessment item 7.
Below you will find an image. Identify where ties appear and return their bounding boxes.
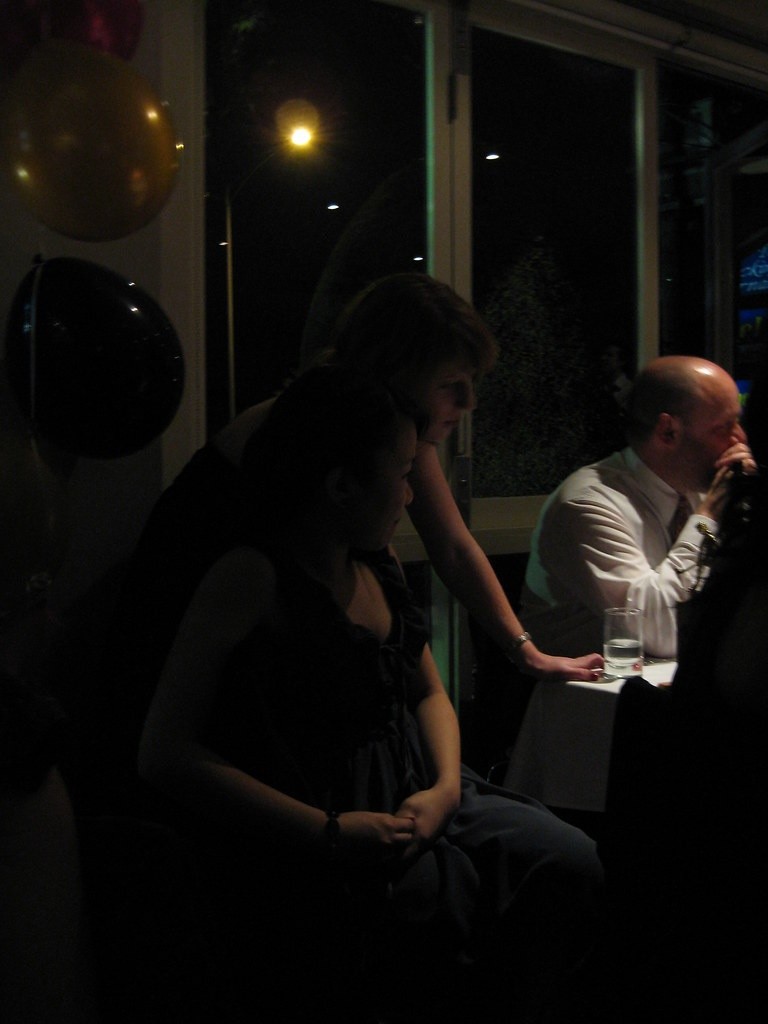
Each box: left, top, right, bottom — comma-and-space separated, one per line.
674, 494, 690, 538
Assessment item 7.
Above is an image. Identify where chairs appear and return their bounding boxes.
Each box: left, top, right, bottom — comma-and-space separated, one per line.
596, 676, 768, 1024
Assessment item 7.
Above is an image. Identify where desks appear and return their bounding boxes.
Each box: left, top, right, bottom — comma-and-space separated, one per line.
502, 658, 678, 827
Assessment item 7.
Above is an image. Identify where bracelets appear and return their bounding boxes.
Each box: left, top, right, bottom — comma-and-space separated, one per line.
326, 807, 340, 847
506, 631, 532, 652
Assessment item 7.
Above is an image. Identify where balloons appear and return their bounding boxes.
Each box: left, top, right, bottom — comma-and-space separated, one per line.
1, 0, 186, 461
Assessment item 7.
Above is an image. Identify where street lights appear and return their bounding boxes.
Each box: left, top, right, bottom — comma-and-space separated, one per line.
222, 98, 324, 436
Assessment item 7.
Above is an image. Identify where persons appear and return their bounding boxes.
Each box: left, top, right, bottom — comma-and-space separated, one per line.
76, 271, 606, 1024
513, 355, 756, 720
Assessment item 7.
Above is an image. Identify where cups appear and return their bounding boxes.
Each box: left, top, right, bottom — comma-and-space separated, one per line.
603, 607, 643, 680
